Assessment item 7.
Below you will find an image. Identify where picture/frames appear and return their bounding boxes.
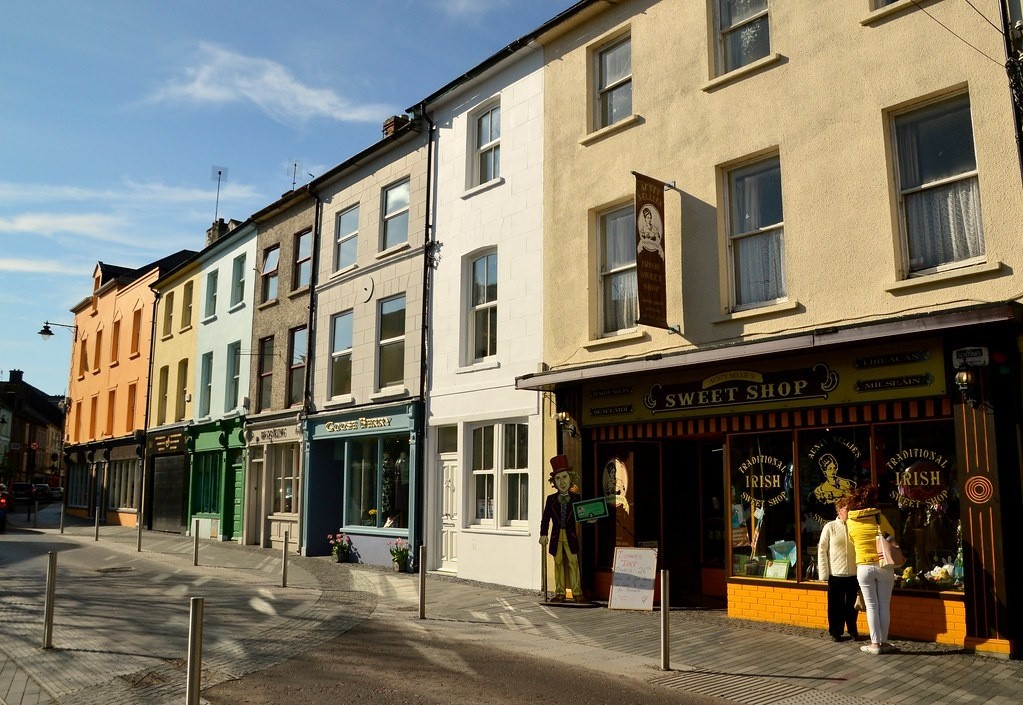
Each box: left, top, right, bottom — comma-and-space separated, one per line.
763, 559, 790, 580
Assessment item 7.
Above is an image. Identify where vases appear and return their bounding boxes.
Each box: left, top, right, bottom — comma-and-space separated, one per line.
372, 517, 376, 526
395, 561, 406, 572
332, 554, 346, 562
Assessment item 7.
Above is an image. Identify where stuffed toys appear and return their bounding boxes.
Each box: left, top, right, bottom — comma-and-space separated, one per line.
902, 566, 913, 579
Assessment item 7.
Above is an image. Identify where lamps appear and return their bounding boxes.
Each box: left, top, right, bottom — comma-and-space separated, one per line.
37, 321, 78, 342
557, 406, 576, 437
953, 355, 980, 408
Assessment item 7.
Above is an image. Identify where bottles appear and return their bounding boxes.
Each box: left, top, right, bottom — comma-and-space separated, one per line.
882, 532, 899, 547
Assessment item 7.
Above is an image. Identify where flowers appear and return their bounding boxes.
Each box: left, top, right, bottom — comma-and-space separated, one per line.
387, 537, 410, 563
368, 509, 377, 517
326, 533, 352, 555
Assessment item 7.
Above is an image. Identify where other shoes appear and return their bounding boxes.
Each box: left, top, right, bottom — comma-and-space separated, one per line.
833, 635, 843, 642
850, 632, 860, 641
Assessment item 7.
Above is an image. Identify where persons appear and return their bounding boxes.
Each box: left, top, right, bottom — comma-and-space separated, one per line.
381, 447, 409, 527
847, 483, 895, 654
817, 495, 862, 642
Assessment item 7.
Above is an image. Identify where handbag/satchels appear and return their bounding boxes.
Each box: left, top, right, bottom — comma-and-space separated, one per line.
854, 590, 866, 612
874, 513, 906, 568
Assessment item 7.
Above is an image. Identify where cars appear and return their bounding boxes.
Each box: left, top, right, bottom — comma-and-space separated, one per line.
50, 486, 64, 501
0, 483, 16, 533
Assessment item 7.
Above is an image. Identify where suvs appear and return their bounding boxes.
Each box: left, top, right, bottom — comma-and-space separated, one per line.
33, 484, 53, 504
8, 481, 40, 507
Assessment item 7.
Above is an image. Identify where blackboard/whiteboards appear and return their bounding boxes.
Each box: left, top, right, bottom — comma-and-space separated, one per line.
608, 547, 658, 610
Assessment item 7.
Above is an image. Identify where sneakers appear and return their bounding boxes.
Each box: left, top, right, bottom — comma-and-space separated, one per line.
860, 645, 881, 654
881, 644, 896, 652
